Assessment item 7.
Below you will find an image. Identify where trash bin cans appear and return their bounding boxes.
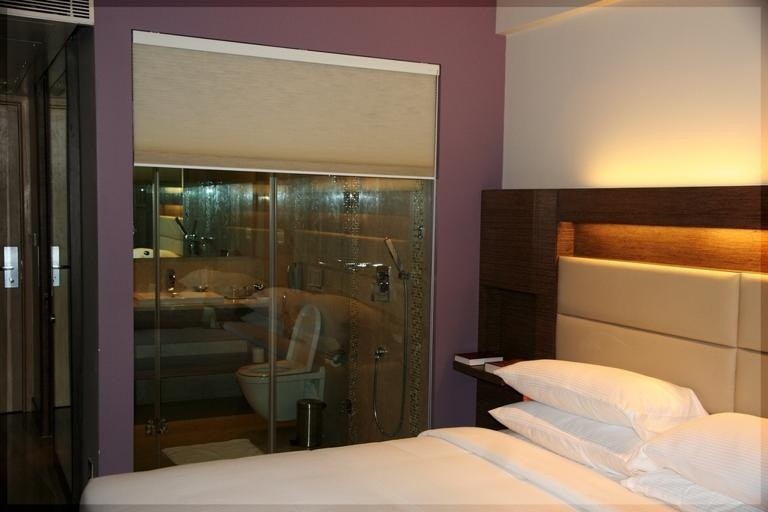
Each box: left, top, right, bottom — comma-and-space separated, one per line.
297, 399, 326, 448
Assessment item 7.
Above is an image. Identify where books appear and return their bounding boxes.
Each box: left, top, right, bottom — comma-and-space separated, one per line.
483, 358, 526, 374
454, 352, 504, 367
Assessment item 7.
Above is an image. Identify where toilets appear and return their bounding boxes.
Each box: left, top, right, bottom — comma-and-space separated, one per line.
236, 305, 327, 422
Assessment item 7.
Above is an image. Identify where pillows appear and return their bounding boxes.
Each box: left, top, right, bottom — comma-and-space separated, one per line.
240, 287, 381, 335
488, 401, 647, 482
178, 268, 265, 299
625, 410, 768, 512
616, 468, 767, 511
493, 358, 711, 442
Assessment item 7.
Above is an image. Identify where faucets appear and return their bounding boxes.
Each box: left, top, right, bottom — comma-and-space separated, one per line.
167, 269, 176, 292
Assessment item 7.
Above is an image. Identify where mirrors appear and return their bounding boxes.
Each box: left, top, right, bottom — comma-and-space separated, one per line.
132, 165, 274, 259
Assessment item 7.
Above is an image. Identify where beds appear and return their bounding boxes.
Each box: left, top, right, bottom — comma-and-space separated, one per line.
131, 203, 185, 258
82, 255, 768, 512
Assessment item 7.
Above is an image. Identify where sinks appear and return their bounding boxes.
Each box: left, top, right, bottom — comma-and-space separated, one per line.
135, 291, 224, 305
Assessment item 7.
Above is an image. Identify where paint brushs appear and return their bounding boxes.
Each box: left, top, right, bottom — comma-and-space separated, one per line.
174, 218, 198, 236
